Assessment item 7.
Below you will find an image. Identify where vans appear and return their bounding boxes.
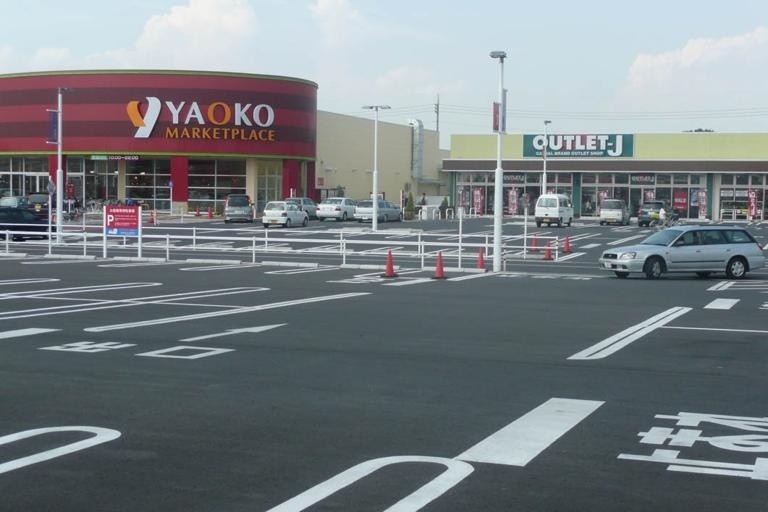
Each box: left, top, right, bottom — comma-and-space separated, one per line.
597, 197, 631, 225
223, 194, 254, 223
533, 192, 574, 227
284, 196, 318, 219
637, 200, 671, 227
352, 199, 404, 223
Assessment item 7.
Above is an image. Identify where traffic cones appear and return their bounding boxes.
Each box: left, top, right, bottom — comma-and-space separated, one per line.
207, 206, 215, 219
541, 238, 554, 260
431, 251, 450, 280
525, 232, 540, 253
147, 209, 154, 224
561, 234, 572, 253
477, 247, 485, 270
193, 206, 202, 217
379, 248, 398, 279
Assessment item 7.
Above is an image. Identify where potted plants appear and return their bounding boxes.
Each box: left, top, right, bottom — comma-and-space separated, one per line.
403, 192, 415, 221
439, 196, 449, 219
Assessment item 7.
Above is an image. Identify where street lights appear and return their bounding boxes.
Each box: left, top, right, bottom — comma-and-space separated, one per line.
541, 119, 553, 197
487, 47, 511, 275
360, 102, 391, 233
52, 85, 72, 246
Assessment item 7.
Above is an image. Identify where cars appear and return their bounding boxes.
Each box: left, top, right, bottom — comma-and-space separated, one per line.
260, 200, 309, 229
314, 197, 358, 221
596, 220, 766, 281
0, 187, 83, 243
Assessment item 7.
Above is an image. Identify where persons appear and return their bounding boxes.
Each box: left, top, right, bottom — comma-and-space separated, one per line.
420, 191, 427, 204
659, 206, 670, 225
649, 212, 659, 228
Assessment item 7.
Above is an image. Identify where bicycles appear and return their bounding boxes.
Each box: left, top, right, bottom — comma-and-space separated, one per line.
646, 211, 680, 234
